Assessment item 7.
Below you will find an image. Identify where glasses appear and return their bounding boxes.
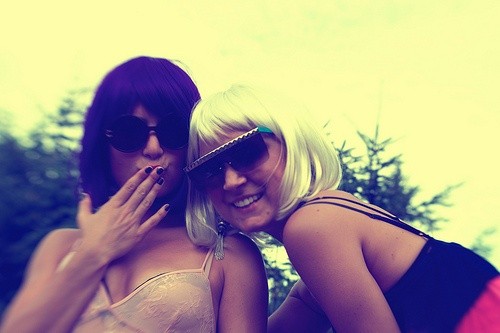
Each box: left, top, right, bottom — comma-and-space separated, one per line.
104, 113, 191, 153
180, 126, 270, 194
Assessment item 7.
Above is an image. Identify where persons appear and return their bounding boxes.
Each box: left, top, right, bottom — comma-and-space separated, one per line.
185, 87, 500, 333
0, 55, 269, 333
